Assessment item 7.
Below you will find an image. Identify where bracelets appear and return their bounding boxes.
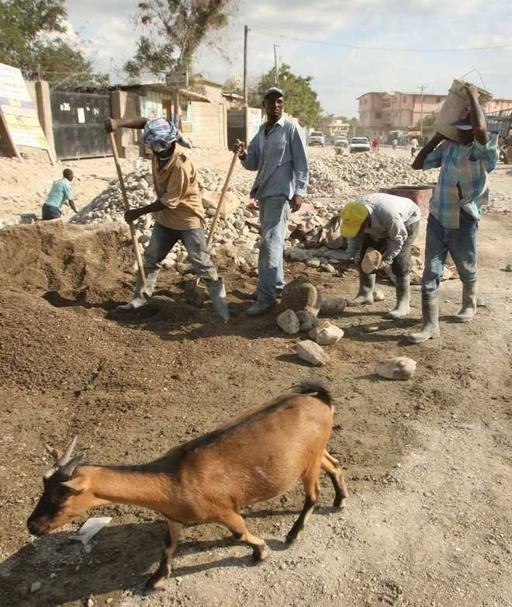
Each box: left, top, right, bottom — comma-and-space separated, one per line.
239, 150, 246, 161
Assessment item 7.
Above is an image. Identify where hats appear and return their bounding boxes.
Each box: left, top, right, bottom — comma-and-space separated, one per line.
264, 86, 285, 100
450, 106, 475, 131
340, 202, 369, 238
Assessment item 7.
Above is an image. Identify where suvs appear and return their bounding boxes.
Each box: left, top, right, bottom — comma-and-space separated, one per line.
349, 137, 372, 156
331, 135, 350, 152
306, 130, 325, 150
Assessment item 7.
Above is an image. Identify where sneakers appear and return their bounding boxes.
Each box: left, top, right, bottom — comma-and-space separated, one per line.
246, 300, 276, 316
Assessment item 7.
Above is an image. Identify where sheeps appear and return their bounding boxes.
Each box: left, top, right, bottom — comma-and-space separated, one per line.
27, 379, 349, 596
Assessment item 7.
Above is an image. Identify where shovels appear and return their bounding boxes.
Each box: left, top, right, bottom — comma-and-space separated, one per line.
109, 131, 160, 315
185, 145, 240, 308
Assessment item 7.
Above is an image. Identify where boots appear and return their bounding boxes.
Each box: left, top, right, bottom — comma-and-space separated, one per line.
206, 277, 229, 323
345, 274, 376, 305
116, 272, 157, 313
386, 274, 411, 319
408, 292, 440, 343
453, 281, 478, 323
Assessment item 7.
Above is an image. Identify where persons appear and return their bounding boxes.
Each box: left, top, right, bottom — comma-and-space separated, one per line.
40, 168, 82, 220
372, 138, 379, 151
408, 80, 499, 346
392, 138, 398, 149
410, 136, 420, 158
104, 115, 234, 321
335, 192, 421, 320
235, 86, 311, 317
